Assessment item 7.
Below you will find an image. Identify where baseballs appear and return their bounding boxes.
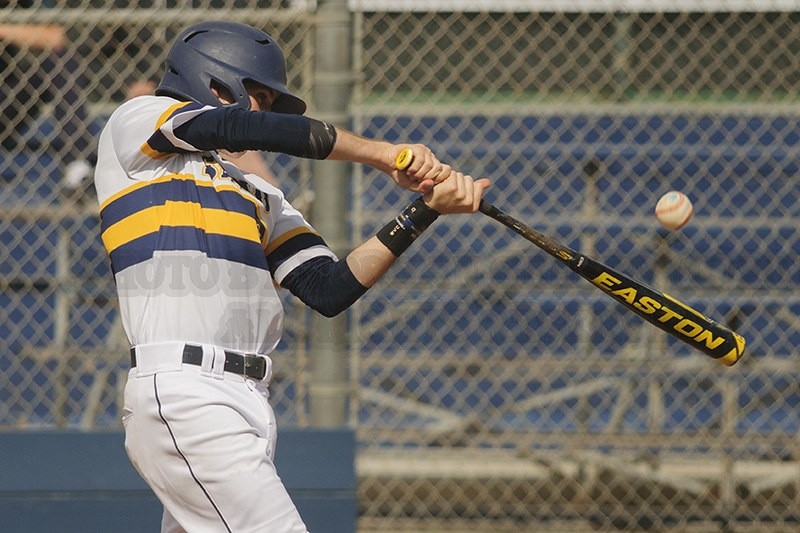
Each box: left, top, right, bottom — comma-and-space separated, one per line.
654, 189, 694, 230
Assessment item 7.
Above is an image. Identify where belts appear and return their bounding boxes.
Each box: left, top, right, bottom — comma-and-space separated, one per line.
129, 342, 267, 381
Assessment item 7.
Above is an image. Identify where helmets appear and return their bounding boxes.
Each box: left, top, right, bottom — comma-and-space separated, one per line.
155, 22, 310, 113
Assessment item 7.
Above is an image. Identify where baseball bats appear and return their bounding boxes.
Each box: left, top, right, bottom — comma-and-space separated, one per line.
395, 149, 746, 368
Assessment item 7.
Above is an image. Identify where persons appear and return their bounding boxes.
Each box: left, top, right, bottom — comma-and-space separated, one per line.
94, 21, 491, 533
